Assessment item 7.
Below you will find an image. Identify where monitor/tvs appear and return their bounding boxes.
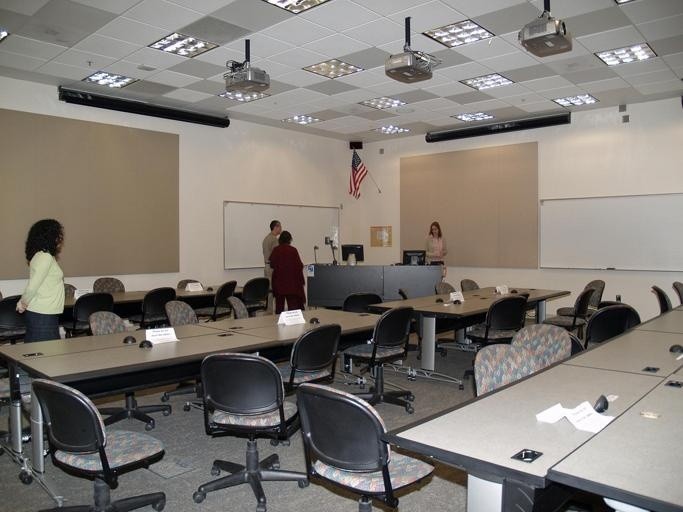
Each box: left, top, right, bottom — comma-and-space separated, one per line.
342, 244, 363, 265
403, 249, 426, 266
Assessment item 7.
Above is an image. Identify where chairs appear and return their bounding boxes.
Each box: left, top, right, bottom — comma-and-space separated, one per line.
93, 278, 126, 294
166, 300, 199, 327
341, 278, 683, 512
129, 286, 178, 328
295, 384, 437, 512
88, 310, 128, 336
229, 295, 250, 319
65, 292, 115, 337
177, 279, 203, 290
1, 293, 27, 343
238, 277, 271, 317
63, 282, 77, 297
194, 280, 238, 321
193, 350, 310, 511
276, 323, 342, 398
30, 377, 171, 511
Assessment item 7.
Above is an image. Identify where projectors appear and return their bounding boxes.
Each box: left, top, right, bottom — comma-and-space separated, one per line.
226, 68, 270, 93
385, 53, 432, 84
518, 12, 572, 58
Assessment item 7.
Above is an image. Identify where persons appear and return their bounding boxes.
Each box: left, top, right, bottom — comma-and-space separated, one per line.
268, 230, 307, 315
424, 221, 449, 279
262, 220, 282, 315
15, 218, 66, 344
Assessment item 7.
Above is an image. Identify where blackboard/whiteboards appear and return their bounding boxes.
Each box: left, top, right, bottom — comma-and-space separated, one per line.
539, 192, 683, 273
223, 200, 341, 271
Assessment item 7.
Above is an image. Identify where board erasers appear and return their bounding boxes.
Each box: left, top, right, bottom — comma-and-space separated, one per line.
607, 268, 615, 270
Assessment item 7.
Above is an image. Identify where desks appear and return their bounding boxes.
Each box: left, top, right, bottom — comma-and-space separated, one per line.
0, 307, 383, 509
64, 284, 244, 336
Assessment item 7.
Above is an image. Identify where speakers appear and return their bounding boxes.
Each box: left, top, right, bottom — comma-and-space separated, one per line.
350, 142, 363, 149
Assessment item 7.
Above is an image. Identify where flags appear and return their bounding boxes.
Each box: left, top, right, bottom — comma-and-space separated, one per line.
348, 149, 369, 201
